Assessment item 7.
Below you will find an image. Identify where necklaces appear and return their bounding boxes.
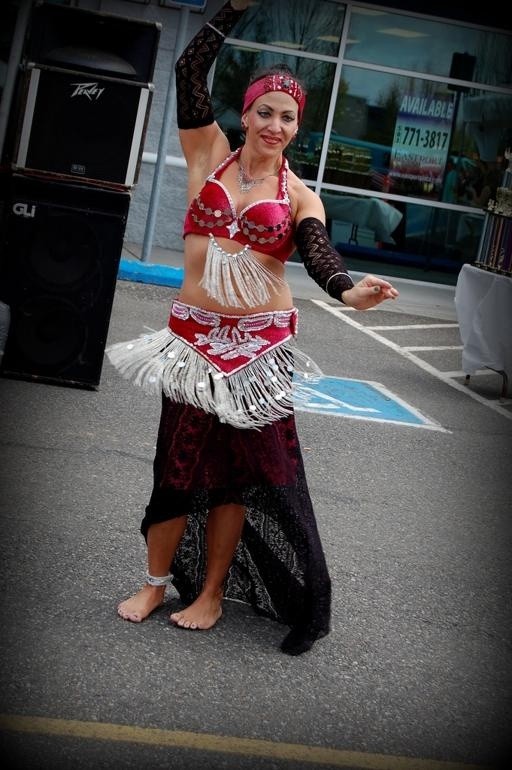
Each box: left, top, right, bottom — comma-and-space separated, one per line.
234, 158, 280, 192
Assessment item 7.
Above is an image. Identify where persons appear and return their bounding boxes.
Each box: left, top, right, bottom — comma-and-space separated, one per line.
109, 0, 399, 631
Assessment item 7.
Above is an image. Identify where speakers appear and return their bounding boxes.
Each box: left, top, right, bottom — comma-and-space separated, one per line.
0, 0, 163, 192
448, 52, 475, 93
0, 173, 131, 391
